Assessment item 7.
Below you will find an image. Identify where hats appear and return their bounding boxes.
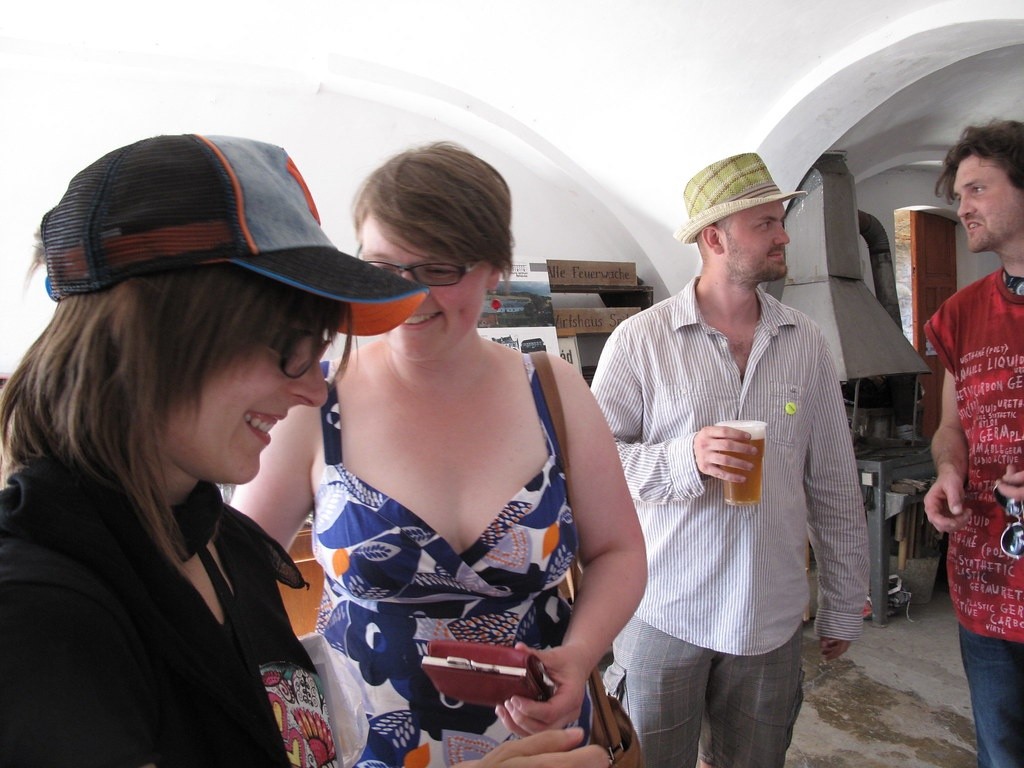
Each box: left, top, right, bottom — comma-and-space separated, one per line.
672, 152, 809, 244
39, 133, 431, 338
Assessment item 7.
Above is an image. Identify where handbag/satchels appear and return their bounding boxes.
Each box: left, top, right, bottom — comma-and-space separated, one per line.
587, 693, 647, 768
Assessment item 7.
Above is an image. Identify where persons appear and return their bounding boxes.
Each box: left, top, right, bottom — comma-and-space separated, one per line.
587, 151, 870, 768
918, 118, 1024, 768
227, 138, 653, 768
0, 132, 611, 768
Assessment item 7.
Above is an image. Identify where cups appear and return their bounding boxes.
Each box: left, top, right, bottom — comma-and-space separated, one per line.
713, 420, 767, 506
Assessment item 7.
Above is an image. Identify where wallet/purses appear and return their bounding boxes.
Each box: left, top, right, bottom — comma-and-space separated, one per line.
421, 641, 550, 707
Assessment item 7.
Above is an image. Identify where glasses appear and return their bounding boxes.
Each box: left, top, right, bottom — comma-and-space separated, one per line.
364, 258, 485, 288
260, 322, 333, 380
